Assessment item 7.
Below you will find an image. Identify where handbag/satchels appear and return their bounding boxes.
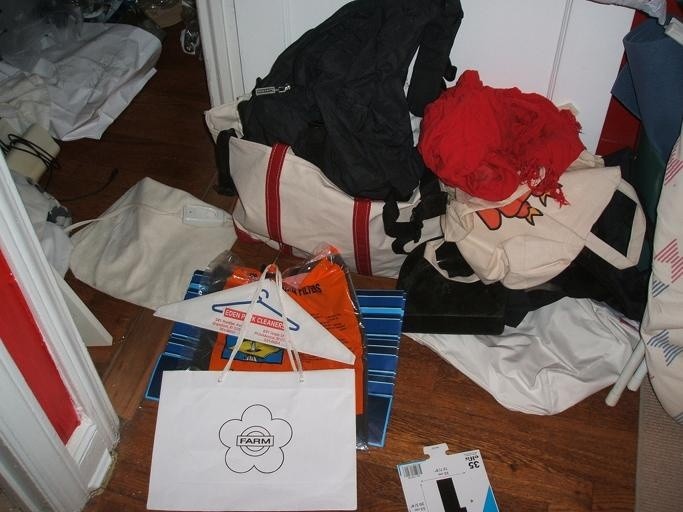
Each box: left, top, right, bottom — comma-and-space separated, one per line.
146, 263, 358, 511
228, 132, 444, 281
447, 163, 646, 291
